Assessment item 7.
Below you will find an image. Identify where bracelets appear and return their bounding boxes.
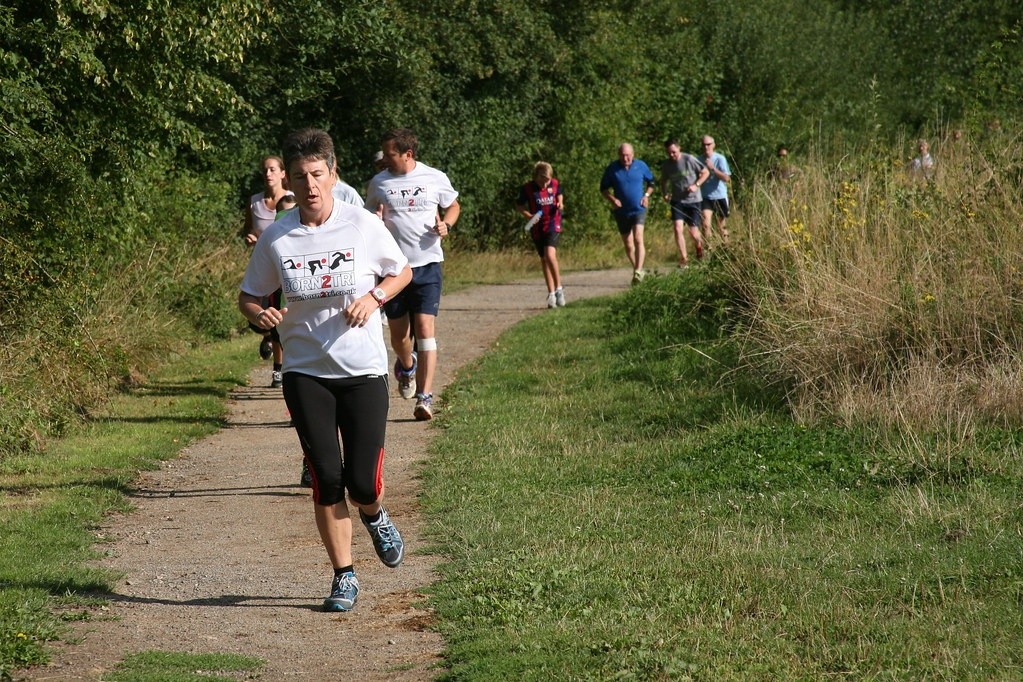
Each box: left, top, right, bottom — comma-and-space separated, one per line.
645, 193, 649, 197
712, 167, 715, 171
256, 310, 264, 319
695, 183, 699, 187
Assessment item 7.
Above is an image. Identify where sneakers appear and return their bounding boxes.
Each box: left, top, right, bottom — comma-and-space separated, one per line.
395, 351, 417, 399
358, 504, 404, 568
414, 394, 433, 420
300, 456, 313, 488
322, 573, 360, 612
259, 336, 273, 360
271, 370, 282, 388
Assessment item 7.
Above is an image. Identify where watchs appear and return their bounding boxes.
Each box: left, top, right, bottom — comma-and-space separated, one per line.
368, 287, 386, 308
447, 223, 451, 233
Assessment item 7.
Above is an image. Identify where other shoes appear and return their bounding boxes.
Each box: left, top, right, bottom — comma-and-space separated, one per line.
632, 270, 645, 285
547, 293, 557, 308
555, 288, 566, 306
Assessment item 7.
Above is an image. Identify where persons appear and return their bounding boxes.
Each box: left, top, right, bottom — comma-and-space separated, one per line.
697, 136, 731, 251
770, 147, 807, 198
332, 181, 365, 207
517, 162, 565, 308
600, 143, 655, 281
368, 129, 460, 420
240, 157, 296, 427
912, 141, 933, 170
239, 131, 414, 613
661, 139, 709, 269
